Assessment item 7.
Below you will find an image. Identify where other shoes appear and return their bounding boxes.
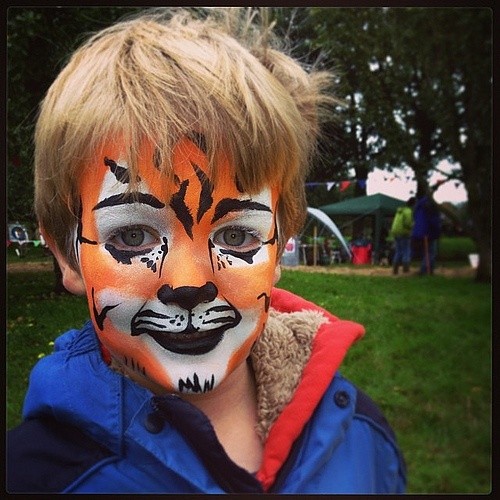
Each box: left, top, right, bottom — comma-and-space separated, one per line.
393, 267, 398, 275
403, 266, 409, 272
415, 271, 432, 278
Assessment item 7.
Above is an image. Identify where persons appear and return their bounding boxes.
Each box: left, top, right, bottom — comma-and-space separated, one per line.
0, 4, 410, 494
